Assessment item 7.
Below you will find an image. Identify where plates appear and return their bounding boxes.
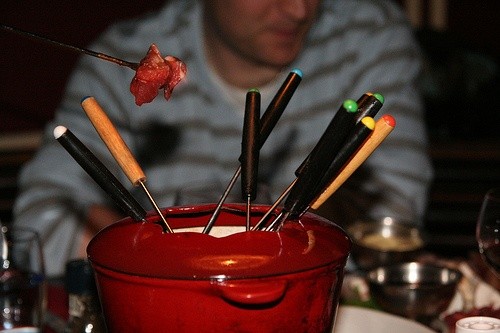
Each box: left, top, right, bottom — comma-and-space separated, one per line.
331, 306, 437, 333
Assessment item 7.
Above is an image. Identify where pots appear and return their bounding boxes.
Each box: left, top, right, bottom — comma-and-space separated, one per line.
86, 202, 352, 333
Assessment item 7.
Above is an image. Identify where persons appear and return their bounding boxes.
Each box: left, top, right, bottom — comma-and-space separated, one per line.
10, 0, 434, 277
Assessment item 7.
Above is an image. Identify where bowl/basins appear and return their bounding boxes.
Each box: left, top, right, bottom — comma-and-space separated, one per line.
365, 259, 464, 326
342, 213, 431, 273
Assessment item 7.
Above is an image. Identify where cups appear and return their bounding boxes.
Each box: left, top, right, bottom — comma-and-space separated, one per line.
475, 193, 500, 279
0, 224, 49, 333
